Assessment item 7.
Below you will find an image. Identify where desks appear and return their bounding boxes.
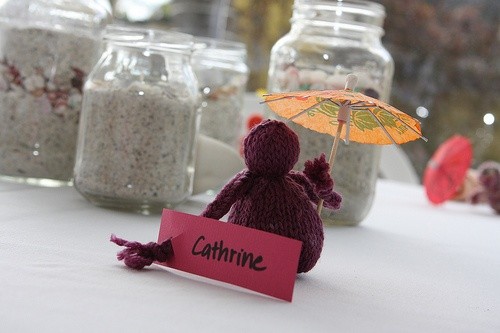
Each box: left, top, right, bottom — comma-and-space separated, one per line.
0, 179, 500, 333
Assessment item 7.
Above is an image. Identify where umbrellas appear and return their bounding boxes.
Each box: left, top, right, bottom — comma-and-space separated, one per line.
421, 132, 482, 204
258, 74, 426, 220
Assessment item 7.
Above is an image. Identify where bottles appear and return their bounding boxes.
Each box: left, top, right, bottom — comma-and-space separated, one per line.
73, 24, 202, 214
191, 37, 250, 188
267, 0, 395, 225
0, 0, 113, 187
